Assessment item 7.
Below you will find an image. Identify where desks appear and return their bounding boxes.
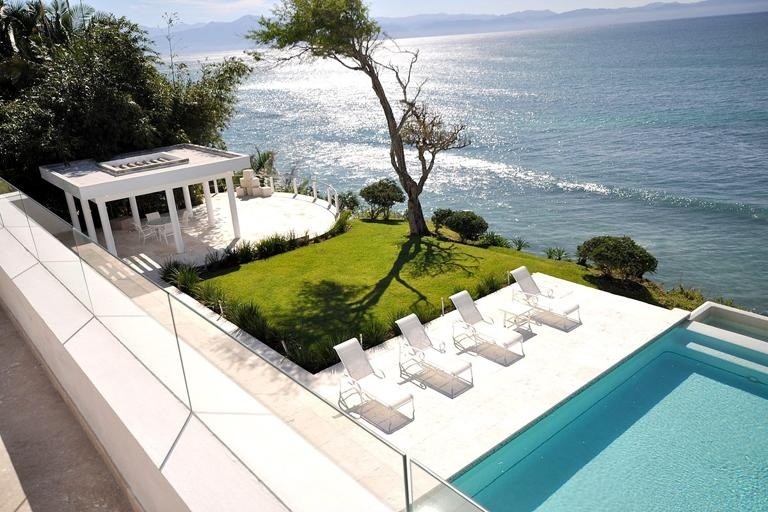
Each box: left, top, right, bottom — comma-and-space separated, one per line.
500, 300, 535, 338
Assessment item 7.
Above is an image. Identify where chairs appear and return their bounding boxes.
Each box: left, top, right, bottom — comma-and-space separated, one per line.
334, 335, 419, 433
393, 312, 477, 401
445, 288, 526, 366
131, 210, 192, 245
509, 265, 581, 333
